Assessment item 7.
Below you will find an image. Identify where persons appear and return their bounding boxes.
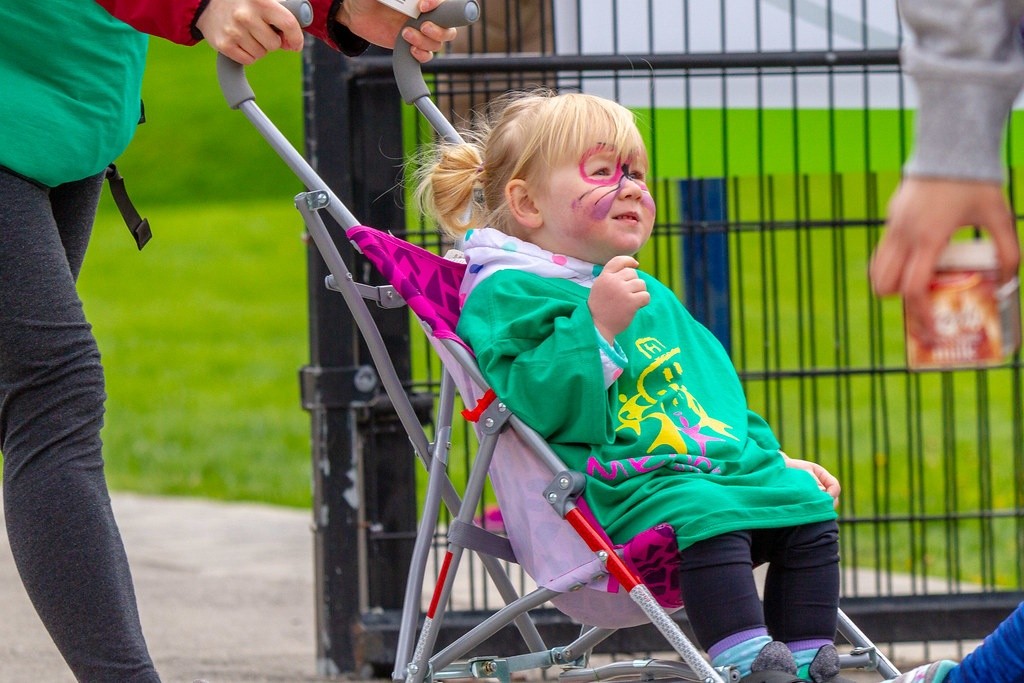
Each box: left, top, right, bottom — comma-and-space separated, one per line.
869, 0, 1024, 683
413, 93, 842, 681
0, 0, 457, 683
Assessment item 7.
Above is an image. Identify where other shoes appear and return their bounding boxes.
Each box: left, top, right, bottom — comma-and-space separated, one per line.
796, 644, 853, 682
742, 641, 801, 682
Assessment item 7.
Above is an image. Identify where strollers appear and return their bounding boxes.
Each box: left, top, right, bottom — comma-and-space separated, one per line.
216, 0, 902, 683
